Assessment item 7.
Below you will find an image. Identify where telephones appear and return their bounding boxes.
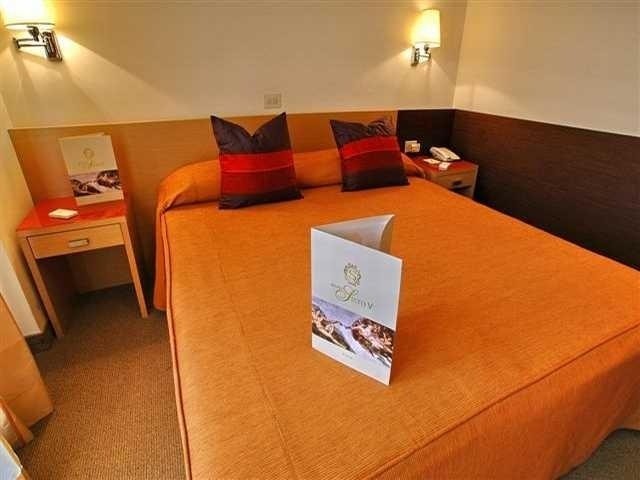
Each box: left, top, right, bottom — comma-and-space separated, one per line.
430, 146, 461, 161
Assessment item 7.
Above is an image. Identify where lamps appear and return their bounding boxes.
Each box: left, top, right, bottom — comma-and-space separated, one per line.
2, 0, 63, 61
412, 9, 442, 66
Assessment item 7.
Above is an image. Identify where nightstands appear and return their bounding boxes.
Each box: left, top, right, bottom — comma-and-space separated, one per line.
417, 153, 476, 196
16, 191, 150, 340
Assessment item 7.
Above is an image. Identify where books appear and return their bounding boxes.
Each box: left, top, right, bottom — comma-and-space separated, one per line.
56, 132, 125, 207
306, 211, 399, 388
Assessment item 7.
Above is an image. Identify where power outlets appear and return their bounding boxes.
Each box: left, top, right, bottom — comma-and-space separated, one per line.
405, 141, 417, 152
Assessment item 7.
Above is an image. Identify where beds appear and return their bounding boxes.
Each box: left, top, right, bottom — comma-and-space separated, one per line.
153, 145, 640, 478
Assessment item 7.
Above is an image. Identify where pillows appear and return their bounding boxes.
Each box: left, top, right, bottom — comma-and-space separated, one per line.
211, 112, 303, 210
329, 116, 409, 193
